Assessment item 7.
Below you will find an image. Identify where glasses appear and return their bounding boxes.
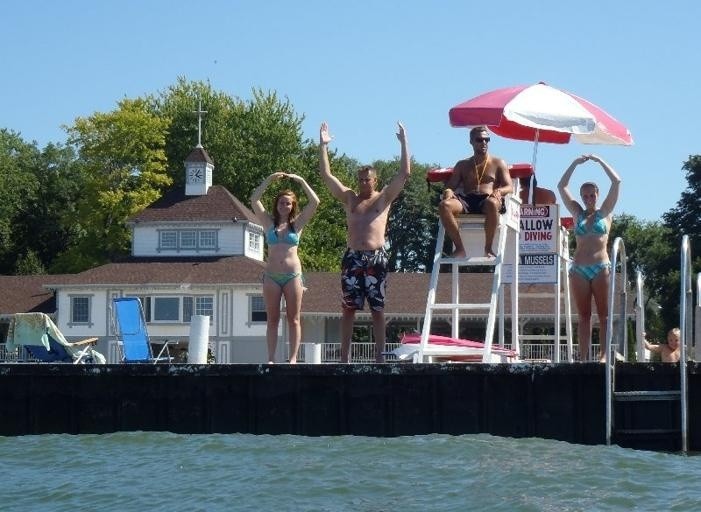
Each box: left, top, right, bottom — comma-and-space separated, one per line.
476, 136, 490, 143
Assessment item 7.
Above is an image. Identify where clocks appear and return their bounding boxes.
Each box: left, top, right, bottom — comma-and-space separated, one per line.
185, 164, 206, 185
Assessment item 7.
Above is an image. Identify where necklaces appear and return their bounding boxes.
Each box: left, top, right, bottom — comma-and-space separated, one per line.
472, 155, 488, 192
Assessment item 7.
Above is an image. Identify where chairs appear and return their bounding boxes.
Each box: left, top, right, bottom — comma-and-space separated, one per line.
9, 297, 180, 365
417, 177, 574, 363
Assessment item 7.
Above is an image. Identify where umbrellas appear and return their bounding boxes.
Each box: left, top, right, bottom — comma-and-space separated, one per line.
447, 81, 634, 207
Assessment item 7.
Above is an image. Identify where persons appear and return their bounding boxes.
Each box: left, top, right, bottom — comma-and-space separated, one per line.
642, 326, 680, 362
248, 172, 320, 365
556, 152, 622, 363
436, 126, 515, 260
318, 120, 411, 363
